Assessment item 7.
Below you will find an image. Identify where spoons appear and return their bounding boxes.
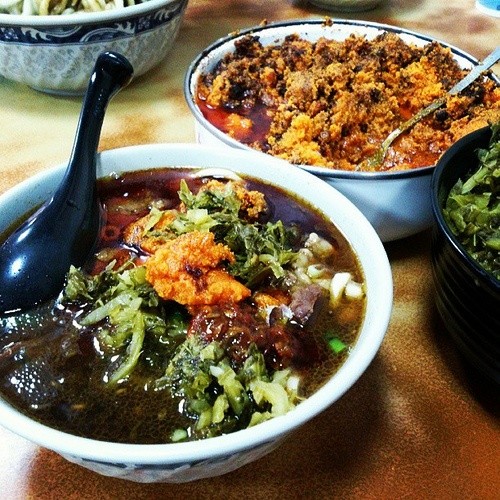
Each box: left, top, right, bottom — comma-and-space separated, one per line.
1, 51, 133, 311
353, 45, 500, 174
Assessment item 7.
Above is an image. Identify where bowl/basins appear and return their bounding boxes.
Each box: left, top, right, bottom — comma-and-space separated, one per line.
0, 144, 393, 481
0, 0, 189, 97
432, 123, 500, 366
185, 19, 500, 242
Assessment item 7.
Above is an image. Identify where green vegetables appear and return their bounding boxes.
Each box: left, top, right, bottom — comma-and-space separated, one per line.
442, 118, 500, 284
